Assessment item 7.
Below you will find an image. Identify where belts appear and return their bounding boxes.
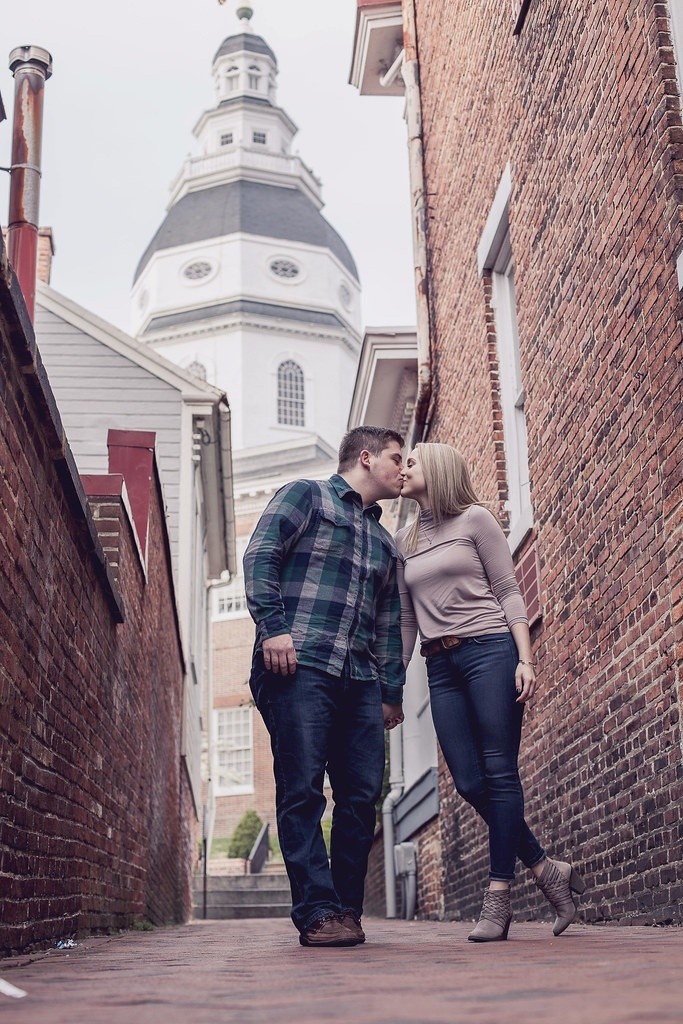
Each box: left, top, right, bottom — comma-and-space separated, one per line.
420, 636, 467, 657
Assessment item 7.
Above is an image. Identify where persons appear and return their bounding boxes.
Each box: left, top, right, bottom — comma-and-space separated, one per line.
243, 426, 406, 946
386, 442, 587, 941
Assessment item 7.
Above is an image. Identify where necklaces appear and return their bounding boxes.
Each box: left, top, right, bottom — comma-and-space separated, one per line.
421, 517, 441, 545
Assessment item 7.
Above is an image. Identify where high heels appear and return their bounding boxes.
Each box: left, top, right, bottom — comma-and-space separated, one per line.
467, 884, 514, 942
535, 855, 587, 936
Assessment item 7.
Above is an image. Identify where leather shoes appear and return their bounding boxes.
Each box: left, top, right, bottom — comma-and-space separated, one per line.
299, 912, 362, 947
338, 910, 366, 945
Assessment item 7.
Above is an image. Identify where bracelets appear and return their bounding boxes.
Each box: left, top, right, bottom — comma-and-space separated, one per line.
519, 660, 537, 668
